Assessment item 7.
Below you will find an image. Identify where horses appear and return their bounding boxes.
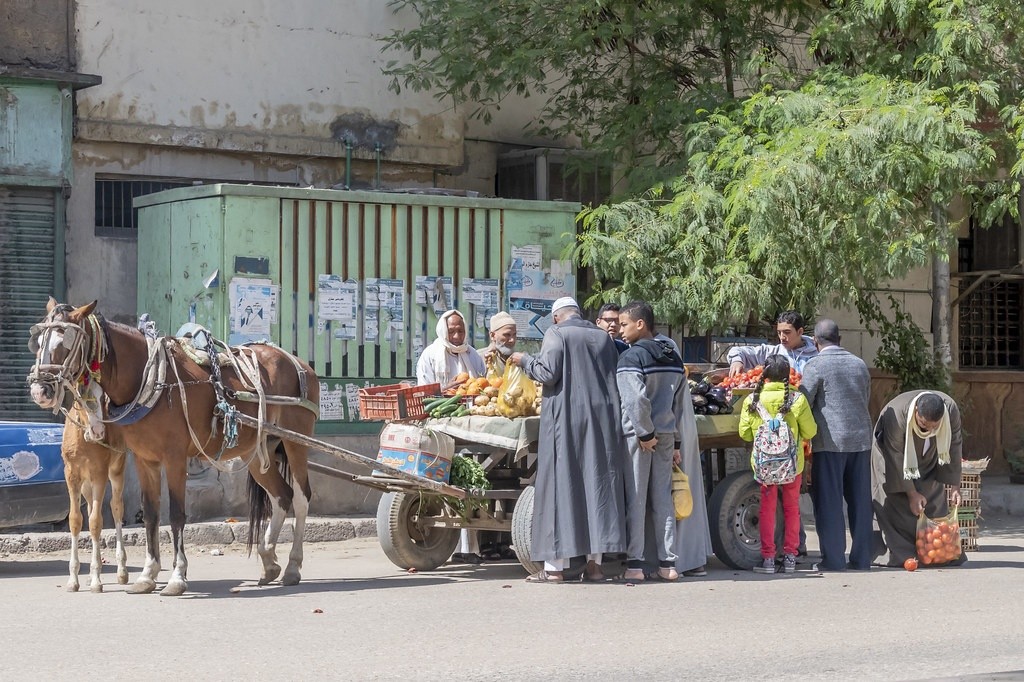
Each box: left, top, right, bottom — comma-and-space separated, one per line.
24, 294, 324, 599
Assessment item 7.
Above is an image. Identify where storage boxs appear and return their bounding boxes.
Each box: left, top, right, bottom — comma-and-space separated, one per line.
359, 383, 440, 420
372, 424, 455, 485
943, 474, 982, 553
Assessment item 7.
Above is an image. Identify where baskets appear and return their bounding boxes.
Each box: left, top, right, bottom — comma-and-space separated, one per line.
357, 383, 441, 421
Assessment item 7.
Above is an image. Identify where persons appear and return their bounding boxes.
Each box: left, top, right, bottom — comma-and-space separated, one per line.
595, 302, 631, 354
474, 311, 518, 560
652, 327, 713, 577
738, 354, 818, 574
798, 318, 872, 572
416, 309, 482, 563
726, 311, 822, 555
511, 296, 627, 584
870, 389, 968, 569
611, 300, 686, 585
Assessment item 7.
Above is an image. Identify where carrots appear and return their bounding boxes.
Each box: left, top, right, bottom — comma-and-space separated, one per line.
444, 373, 503, 394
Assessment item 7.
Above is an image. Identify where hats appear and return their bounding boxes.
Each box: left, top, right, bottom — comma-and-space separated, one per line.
489, 310, 516, 332
552, 296, 578, 313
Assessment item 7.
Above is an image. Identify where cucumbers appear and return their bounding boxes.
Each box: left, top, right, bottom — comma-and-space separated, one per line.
421, 394, 470, 417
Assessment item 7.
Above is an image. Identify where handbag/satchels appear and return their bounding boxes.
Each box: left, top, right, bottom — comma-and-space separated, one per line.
496, 355, 536, 419
669, 460, 693, 519
915, 501, 962, 564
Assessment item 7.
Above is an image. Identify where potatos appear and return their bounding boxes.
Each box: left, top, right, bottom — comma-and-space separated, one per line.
470, 382, 543, 418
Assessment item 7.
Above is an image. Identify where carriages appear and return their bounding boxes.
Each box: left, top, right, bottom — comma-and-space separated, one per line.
23, 294, 784, 597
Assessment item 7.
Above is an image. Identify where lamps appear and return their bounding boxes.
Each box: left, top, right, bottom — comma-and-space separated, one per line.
59, 82, 71, 99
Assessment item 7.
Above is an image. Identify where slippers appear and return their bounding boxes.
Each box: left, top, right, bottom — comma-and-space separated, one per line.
612, 569, 644, 584
576, 568, 604, 582
479, 547, 501, 560
496, 542, 517, 559
646, 571, 678, 583
683, 567, 706, 576
525, 568, 566, 584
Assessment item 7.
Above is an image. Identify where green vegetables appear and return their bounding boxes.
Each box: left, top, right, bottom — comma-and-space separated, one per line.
449, 456, 492, 522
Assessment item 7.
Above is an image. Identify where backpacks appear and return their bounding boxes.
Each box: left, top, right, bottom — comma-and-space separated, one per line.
749, 391, 801, 486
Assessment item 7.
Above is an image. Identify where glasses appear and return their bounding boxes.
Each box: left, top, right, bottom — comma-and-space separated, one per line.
599, 317, 619, 326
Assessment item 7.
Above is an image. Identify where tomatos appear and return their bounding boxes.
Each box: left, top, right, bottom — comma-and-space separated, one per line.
915, 523, 961, 564
717, 365, 802, 388
903, 558, 917, 570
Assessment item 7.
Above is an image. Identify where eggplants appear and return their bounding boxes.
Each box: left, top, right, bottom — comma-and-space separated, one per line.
690, 382, 734, 416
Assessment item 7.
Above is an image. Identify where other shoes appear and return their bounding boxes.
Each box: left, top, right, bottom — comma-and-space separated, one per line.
782, 553, 796, 573
452, 551, 483, 564
847, 560, 855, 569
776, 552, 808, 563
753, 557, 775, 574
811, 562, 820, 570
